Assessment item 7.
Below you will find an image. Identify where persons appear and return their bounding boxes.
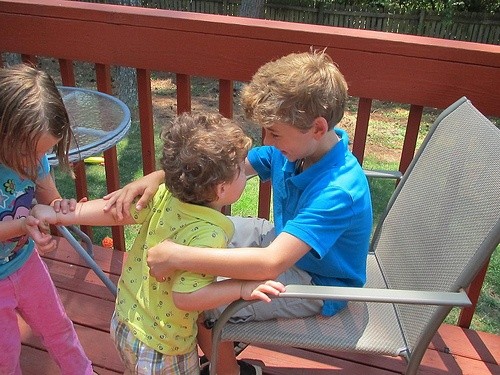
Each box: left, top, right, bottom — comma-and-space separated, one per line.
0, 64, 95, 374
102, 46, 374, 375
30, 110, 286, 374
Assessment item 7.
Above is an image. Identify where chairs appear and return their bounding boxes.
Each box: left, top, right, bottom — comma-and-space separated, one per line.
210, 96, 500, 375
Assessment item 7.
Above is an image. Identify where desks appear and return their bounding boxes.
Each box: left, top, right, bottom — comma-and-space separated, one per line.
46, 86, 130, 298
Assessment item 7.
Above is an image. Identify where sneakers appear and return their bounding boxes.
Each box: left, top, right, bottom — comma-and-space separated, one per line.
199, 341, 262, 375
